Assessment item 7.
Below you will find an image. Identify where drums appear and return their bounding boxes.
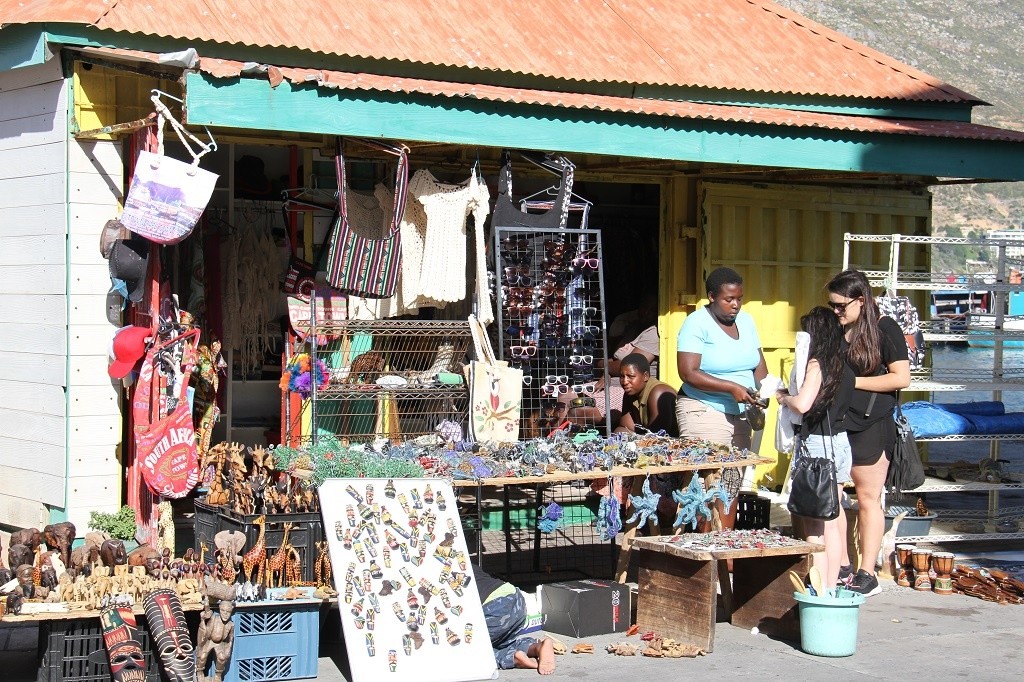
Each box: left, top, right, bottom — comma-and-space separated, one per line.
911, 548, 934, 591
931, 551, 956, 595
896, 544, 916, 587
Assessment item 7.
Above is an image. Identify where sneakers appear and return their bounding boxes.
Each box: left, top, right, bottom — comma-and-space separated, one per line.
849, 570, 883, 597
837, 566, 854, 583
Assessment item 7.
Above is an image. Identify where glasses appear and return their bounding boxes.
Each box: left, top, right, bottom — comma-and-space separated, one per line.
501, 237, 597, 406
827, 298, 858, 311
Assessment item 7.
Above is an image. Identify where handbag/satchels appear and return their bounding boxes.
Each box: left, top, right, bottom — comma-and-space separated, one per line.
884, 414, 925, 501
324, 150, 408, 298
135, 402, 198, 499
122, 118, 221, 245
787, 457, 839, 520
463, 313, 523, 447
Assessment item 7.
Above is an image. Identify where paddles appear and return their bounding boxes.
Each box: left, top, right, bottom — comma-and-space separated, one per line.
951, 563, 1024, 606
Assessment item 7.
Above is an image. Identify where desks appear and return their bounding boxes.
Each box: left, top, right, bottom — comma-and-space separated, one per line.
626, 534, 826, 656
449, 452, 777, 621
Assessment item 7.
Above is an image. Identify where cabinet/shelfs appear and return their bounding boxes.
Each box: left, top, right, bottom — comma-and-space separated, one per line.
162, 137, 304, 445
844, 231, 1024, 580
286, 288, 479, 447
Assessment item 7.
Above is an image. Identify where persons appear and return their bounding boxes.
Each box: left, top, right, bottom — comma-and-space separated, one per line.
676, 268, 769, 572
614, 353, 679, 439
777, 307, 853, 587
14, 563, 41, 599
197, 600, 235, 682
557, 288, 659, 425
817, 270, 910, 597
471, 563, 555, 675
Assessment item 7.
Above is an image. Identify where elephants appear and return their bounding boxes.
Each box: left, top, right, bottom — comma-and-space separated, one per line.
0, 521, 202, 617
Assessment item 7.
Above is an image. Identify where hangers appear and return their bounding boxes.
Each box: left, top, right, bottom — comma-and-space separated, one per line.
344, 135, 411, 156
422, 151, 473, 186
518, 178, 593, 211
276, 174, 339, 214
150, 89, 218, 152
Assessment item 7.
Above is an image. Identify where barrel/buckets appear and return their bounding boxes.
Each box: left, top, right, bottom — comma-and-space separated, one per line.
792, 588, 866, 657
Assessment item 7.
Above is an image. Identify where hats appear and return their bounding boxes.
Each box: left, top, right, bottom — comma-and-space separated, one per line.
109, 327, 150, 378
109, 239, 150, 301
100, 220, 130, 260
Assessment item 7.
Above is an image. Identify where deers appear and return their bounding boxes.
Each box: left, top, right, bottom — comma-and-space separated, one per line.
219, 513, 332, 590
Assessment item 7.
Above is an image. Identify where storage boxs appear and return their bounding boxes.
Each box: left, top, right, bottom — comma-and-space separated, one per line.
542, 578, 632, 639
884, 510, 939, 536
36, 497, 338, 681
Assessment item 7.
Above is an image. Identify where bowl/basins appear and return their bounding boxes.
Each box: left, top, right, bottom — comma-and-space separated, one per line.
884, 505, 939, 537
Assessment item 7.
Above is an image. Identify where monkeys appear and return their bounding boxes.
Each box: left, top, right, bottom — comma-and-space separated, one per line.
151, 546, 213, 587
